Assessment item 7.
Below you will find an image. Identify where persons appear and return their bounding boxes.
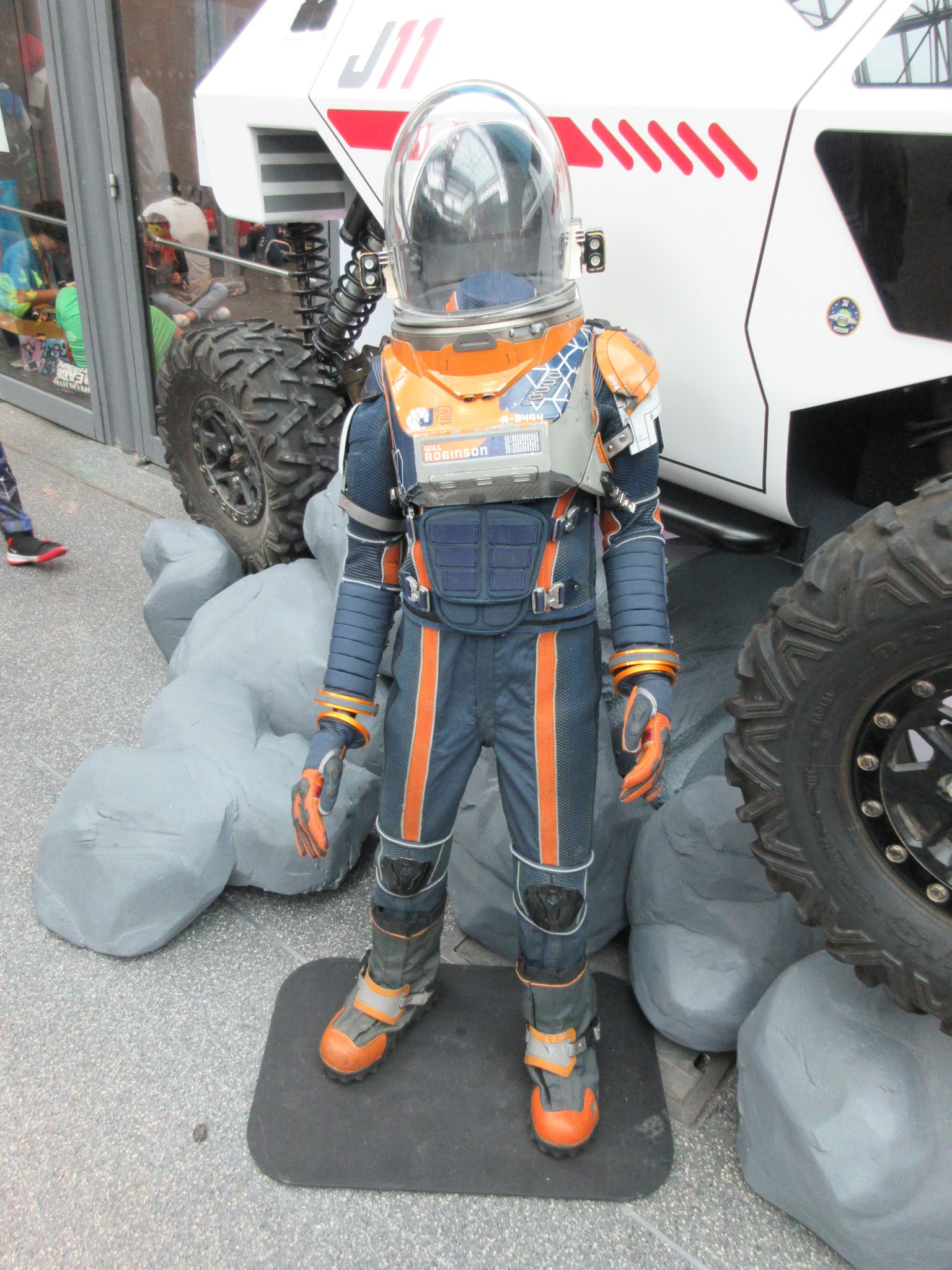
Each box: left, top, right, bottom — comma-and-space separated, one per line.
0, 444, 70, 565
291, 84, 682, 1157
1, 15, 296, 426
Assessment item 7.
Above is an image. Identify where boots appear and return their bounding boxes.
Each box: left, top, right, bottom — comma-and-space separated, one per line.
516, 959, 601, 1158
318, 907, 448, 1084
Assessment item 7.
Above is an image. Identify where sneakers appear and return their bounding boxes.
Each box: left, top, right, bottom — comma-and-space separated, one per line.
172, 314, 190, 328
205, 307, 230, 323
6, 533, 67, 566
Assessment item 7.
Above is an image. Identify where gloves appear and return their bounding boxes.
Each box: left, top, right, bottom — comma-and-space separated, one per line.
290, 717, 356, 860
618, 674, 672, 805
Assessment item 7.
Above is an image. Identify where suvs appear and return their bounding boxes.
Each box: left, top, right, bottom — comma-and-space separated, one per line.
159, 0, 952, 1038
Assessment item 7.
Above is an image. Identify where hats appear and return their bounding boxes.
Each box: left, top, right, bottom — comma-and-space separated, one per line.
15, 34, 45, 72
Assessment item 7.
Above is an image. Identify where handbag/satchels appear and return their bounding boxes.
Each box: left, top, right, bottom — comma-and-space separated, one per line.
9, 94, 36, 179
